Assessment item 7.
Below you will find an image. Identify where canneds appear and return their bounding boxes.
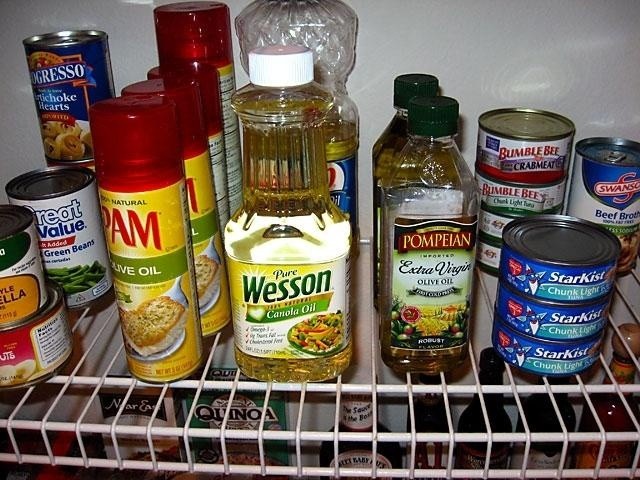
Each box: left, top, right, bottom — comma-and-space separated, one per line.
491, 214, 622, 374
5, 165, 113, 309
0, 277, 74, 391
1, 204, 50, 328
45, 153, 95, 172
474, 108, 576, 278
22, 30, 117, 161
566, 137, 640, 272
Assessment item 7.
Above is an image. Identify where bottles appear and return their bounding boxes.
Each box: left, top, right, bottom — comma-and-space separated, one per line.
89, 2, 475, 384
318, 324, 639, 478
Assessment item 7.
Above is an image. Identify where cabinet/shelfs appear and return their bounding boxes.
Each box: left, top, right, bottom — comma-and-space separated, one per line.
0, 237, 640, 478
